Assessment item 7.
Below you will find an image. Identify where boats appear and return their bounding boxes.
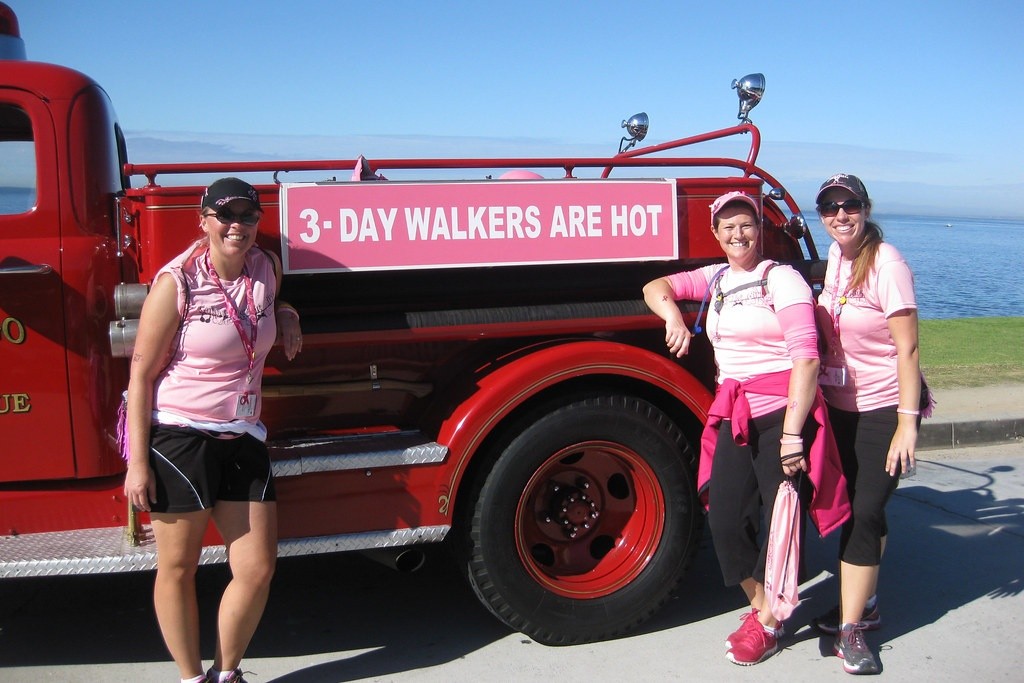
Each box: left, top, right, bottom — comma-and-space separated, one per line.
947, 223, 953, 227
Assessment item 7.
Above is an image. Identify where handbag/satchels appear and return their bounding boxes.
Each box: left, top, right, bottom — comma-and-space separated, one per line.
115, 399, 131, 469
764, 481, 800, 621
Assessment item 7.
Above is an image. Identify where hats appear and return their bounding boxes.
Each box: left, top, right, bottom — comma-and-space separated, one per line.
200, 177, 264, 215
815, 173, 868, 205
709, 190, 760, 226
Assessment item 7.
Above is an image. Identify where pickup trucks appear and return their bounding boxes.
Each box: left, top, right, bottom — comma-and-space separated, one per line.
0, 1, 829, 648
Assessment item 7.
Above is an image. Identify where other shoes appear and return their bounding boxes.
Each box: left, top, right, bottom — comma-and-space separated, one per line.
199, 667, 258, 683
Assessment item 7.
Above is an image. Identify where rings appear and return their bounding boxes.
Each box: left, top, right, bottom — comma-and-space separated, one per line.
297, 337, 300, 341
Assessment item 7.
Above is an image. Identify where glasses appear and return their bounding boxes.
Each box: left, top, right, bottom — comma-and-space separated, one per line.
203, 210, 260, 226
816, 199, 868, 216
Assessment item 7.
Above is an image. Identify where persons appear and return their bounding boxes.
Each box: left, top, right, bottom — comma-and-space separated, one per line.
640, 189, 822, 666
812, 173, 925, 674
122, 177, 303, 683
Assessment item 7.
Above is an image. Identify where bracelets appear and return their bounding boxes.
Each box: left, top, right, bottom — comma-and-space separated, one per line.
896, 408, 920, 415
778, 432, 805, 467
275, 303, 300, 320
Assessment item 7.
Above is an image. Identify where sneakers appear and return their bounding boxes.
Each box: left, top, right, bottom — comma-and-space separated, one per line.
726, 620, 777, 666
833, 620, 883, 675
725, 608, 785, 650
810, 598, 881, 635
